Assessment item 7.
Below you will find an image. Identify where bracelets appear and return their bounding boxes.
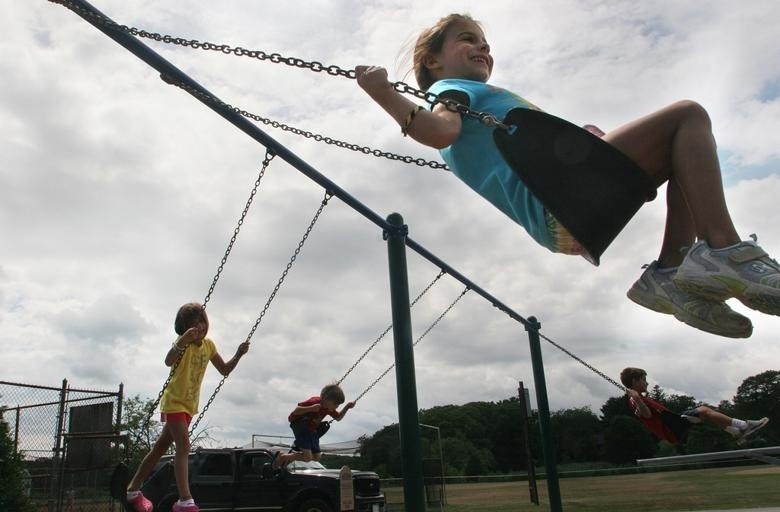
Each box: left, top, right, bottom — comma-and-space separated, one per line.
400, 106, 426, 138
171, 340, 186, 352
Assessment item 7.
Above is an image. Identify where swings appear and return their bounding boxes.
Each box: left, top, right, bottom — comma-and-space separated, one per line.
109, 149, 332, 508
60, 1, 657, 267
286, 270, 470, 443
495, 305, 697, 444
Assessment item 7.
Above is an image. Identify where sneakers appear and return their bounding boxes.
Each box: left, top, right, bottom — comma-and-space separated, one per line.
626, 260, 753, 340
672, 240, 780, 317
733, 417, 770, 445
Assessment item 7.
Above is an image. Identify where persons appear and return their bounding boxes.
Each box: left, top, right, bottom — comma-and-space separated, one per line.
113, 454, 135, 512
270, 383, 355, 470
64, 487, 74, 511
116, 301, 251, 511
351, 11, 779, 339
618, 367, 770, 446
13, 461, 33, 512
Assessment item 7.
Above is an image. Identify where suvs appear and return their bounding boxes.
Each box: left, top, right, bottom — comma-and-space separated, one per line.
140, 446, 387, 512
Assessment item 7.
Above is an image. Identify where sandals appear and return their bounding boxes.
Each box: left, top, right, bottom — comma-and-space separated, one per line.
271, 449, 294, 470
126, 489, 154, 512
172, 499, 198, 512
